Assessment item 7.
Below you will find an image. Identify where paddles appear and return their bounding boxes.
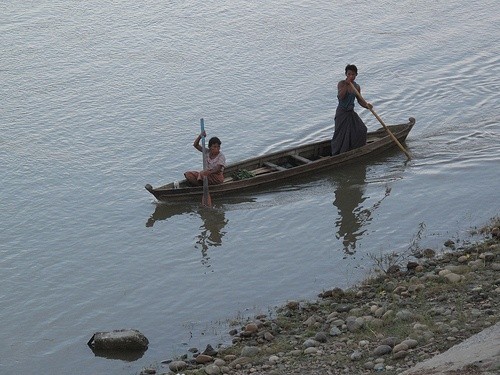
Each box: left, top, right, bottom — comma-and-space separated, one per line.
347, 79, 412, 161
199, 118, 211, 207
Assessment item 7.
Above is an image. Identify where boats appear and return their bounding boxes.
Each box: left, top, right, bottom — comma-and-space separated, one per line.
144, 117, 417, 203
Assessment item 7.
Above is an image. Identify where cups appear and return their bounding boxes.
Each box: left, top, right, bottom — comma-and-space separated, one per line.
174, 180, 179, 189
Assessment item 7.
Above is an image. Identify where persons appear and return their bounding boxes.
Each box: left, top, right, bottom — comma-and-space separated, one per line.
184, 131, 225, 185
331, 64, 373, 156
